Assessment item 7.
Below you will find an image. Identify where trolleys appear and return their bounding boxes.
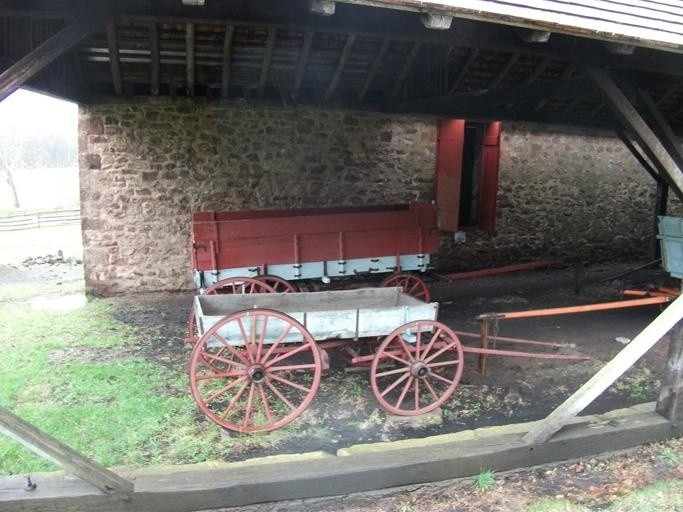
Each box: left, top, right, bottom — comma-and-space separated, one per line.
189, 201, 565, 303
187, 271, 587, 433
